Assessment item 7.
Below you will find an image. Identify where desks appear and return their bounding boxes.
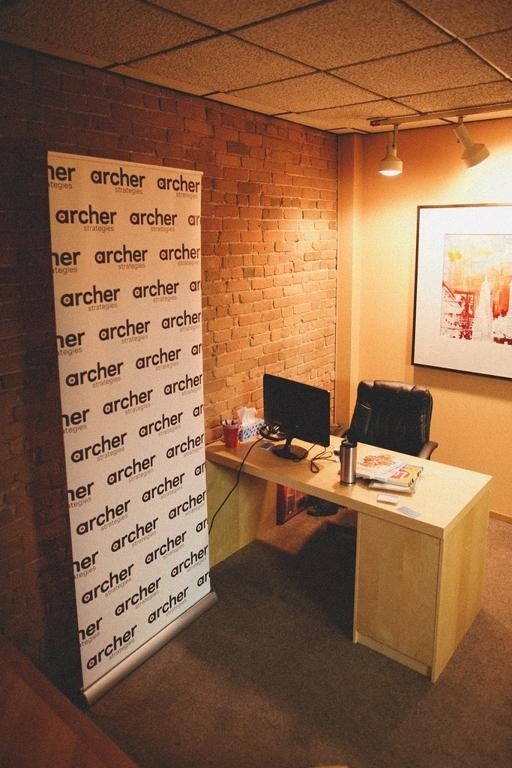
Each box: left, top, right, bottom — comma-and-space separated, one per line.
207, 433, 493, 683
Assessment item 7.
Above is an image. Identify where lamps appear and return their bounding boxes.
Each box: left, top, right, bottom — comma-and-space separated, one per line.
370, 101, 512, 176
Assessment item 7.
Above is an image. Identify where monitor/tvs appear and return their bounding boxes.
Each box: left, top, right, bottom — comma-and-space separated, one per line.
261, 372, 330, 462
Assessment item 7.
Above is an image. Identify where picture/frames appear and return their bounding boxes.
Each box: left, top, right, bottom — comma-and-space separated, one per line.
411, 205, 512, 380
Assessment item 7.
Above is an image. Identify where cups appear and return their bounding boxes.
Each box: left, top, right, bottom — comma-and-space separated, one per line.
340, 438, 358, 487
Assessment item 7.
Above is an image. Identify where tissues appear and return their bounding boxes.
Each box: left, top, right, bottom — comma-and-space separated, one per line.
236, 406, 265, 442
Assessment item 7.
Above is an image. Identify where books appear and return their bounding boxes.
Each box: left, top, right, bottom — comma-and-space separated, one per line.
338, 452, 408, 483
378, 463, 424, 487
367, 472, 420, 496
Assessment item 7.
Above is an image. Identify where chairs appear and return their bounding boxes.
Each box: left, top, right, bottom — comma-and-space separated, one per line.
341, 380, 438, 461
